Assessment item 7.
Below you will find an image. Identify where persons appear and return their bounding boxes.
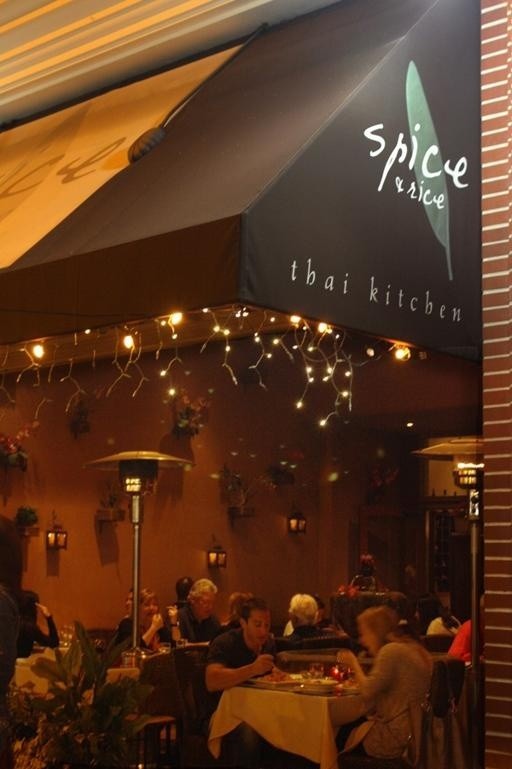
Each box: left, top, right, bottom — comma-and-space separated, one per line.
175, 578, 222, 642
282, 596, 325, 637
284, 592, 339, 640
337, 604, 435, 759
125, 588, 141, 613
10, 591, 61, 658
339, 544, 380, 592
385, 590, 419, 645
1, 515, 22, 769
446, 591, 484, 667
412, 593, 461, 635
199, 597, 279, 769
210, 591, 254, 645
115, 587, 182, 650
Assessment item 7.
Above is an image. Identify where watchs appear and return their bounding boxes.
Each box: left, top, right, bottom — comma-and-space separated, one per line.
170, 620, 181, 627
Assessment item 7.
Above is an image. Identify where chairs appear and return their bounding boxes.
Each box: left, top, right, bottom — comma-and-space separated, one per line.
344, 654, 467, 769
173, 643, 241, 769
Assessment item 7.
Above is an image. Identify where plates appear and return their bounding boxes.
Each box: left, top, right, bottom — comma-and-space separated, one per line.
297, 678, 339, 692
247, 674, 304, 686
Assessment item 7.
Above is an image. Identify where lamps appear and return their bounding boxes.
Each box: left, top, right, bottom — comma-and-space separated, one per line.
208, 542, 227, 568
48, 510, 68, 550
289, 511, 307, 533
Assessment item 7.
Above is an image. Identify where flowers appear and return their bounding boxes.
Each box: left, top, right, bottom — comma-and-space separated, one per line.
0, 432, 34, 468
175, 386, 212, 437
